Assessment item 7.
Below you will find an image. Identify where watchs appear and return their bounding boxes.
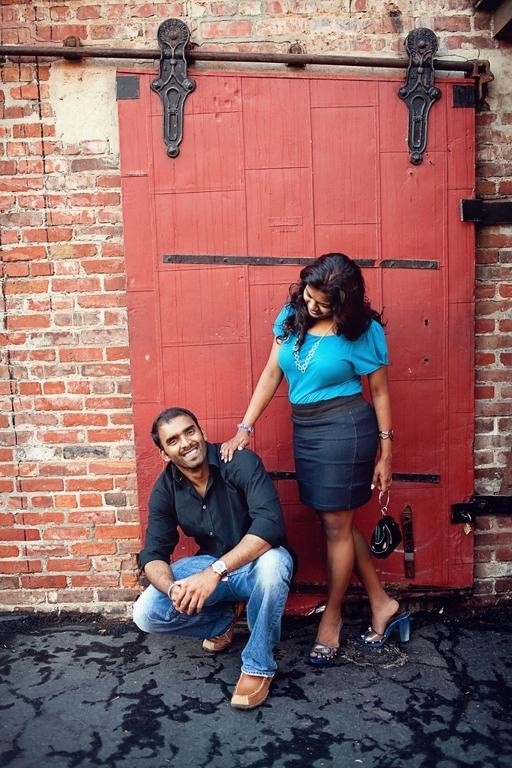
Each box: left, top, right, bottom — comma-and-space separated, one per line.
210, 559, 228, 577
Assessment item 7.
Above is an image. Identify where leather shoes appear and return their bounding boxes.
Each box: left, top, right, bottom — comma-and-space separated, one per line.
231, 671, 273, 710
202, 602, 245, 653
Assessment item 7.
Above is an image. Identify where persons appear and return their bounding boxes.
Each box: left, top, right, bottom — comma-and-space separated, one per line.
132, 407, 299, 711
220, 252, 411, 666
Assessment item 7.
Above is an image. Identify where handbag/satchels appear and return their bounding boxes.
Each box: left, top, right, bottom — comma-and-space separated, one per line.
371, 489, 401, 558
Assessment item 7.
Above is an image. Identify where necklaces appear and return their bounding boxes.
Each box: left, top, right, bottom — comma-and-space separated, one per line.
292, 319, 335, 373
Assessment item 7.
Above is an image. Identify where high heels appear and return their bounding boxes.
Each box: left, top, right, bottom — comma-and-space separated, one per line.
356, 611, 412, 648
309, 619, 346, 665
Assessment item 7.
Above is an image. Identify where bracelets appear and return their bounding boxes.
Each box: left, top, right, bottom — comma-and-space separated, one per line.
377, 428, 396, 442
167, 583, 176, 599
236, 423, 254, 433
